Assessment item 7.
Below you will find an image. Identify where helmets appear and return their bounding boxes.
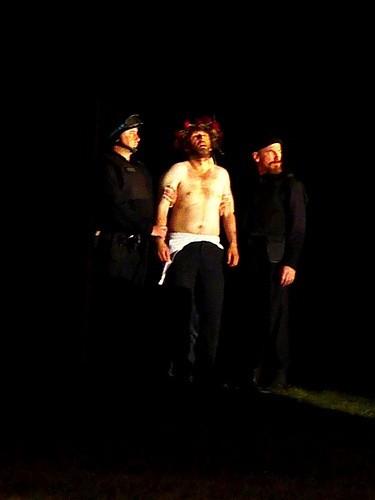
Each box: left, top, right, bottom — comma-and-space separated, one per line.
108, 113, 143, 140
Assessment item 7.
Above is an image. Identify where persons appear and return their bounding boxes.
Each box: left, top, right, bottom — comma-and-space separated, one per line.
89, 111, 176, 322
150, 114, 241, 363
219, 137, 306, 388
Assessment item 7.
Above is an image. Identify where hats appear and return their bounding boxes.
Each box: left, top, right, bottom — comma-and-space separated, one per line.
247, 133, 282, 154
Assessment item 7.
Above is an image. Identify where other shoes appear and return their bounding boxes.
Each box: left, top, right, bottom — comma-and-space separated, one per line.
272, 373, 286, 387
